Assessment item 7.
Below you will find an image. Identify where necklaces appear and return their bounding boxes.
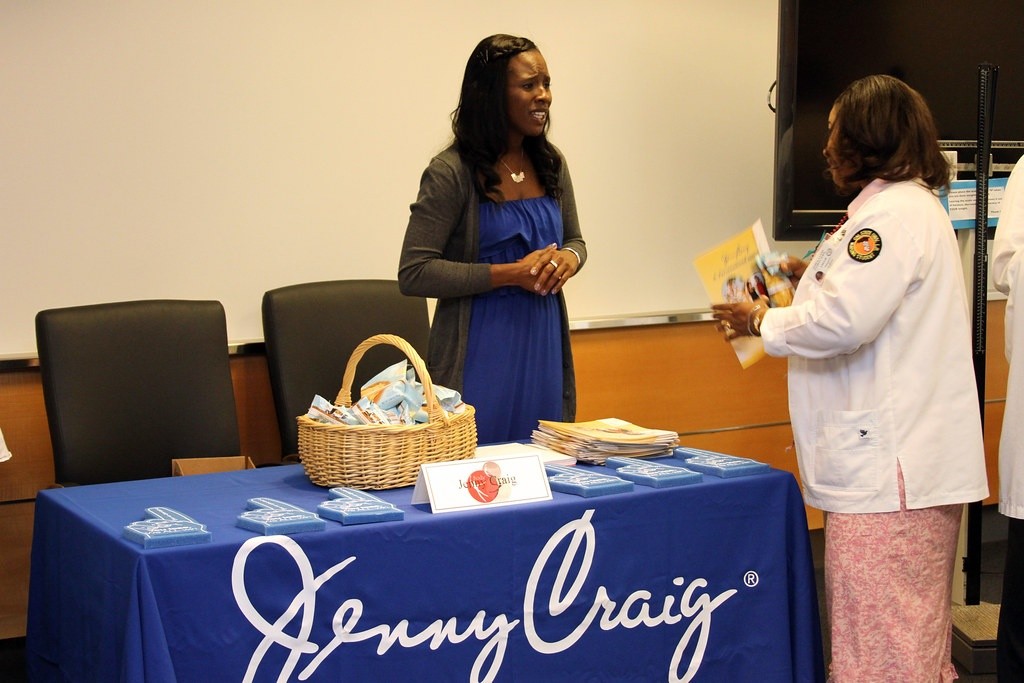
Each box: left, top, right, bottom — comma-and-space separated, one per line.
501, 148, 525, 183
808, 212, 848, 264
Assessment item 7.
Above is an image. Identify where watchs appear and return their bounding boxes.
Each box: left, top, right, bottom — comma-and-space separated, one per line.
753, 309, 764, 332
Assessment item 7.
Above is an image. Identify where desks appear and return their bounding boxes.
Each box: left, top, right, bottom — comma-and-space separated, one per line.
22, 438, 827, 683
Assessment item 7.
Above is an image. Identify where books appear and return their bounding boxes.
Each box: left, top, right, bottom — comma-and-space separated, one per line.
476, 443, 577, 466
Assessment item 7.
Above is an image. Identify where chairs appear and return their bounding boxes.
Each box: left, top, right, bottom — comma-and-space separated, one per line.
34, 299, 241, 491
261, 277, 431, 465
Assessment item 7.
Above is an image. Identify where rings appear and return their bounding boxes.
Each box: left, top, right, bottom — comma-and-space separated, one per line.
721, 320, 730, 328
549, 261, 558, 268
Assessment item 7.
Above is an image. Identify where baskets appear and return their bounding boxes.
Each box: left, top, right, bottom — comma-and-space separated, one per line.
296, 333, 477, 489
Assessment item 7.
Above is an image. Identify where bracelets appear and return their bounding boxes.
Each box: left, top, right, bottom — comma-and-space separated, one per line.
561, 248, 580, 263
747, 304, 761, 336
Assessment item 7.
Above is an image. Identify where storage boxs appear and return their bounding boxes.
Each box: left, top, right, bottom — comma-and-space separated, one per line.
171, 455, 258, 476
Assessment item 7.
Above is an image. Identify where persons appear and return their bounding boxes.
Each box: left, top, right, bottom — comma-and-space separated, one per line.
397, 34, 588, 444
713, 75, 991, 683
725, 281, 738, 304
746, 275, 765, 301
991, 155, 1024, 683
815, 271, 824, 282
